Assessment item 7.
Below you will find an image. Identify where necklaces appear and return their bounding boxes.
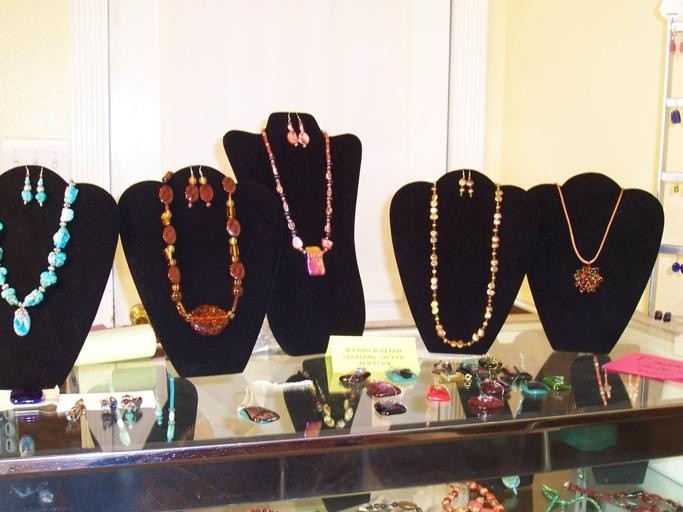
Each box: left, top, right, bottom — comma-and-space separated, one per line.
358, 476, 683, 512
553, 179, 629, 299
591, 357, 618, 409
300, 371, 357, 429
105, 374, 176, 449
4, 408, 56, 504
159, 170, 245, 340
427, 177, 505, 351
255, 126, 349, 282
0, 178, 84, 344
